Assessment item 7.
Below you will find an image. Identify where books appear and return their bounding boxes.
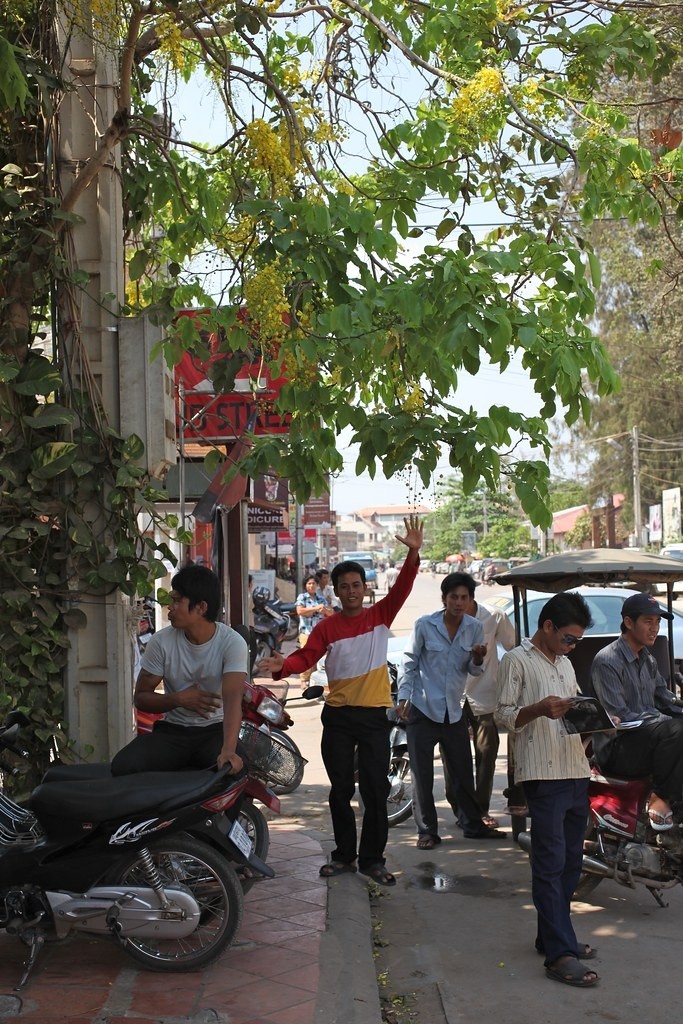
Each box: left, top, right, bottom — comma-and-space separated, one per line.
558, 696, 644, 737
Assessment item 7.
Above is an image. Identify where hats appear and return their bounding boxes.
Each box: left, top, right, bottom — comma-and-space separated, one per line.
621, 594, 674, 620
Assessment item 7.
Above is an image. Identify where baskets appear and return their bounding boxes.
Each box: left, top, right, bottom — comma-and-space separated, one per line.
237, 721, 308, 787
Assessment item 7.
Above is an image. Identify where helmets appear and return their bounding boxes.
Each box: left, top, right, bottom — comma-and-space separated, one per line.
254, 586, 270, 605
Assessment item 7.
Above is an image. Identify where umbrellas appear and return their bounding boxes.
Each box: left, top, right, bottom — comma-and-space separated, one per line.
446, 554, 463, 562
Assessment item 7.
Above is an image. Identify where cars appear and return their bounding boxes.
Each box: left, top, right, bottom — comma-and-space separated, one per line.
395, 559, 531, 587
660, 543, 683, 561
308, 584, 683, 741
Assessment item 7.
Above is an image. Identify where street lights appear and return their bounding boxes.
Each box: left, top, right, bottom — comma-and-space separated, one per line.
605, 424, 642, 547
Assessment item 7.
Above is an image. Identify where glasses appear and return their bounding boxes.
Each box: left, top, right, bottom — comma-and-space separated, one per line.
553, 624, 582, 644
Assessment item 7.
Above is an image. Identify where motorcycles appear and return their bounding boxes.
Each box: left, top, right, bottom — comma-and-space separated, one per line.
503, 670, 683, 908
0, 588, 326, 992
359, 660, 414, 828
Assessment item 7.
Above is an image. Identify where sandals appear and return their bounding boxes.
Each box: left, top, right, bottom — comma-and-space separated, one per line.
545, 957, 601, 988
538, 943, 597, 959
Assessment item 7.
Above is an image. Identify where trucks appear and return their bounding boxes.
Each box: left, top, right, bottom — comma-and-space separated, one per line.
337, 551, 378, 589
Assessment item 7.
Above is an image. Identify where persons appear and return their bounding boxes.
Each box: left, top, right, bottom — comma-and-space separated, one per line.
396, 571, 683, 987
431, 563, 453, 577
479, 559, 495, 580
257, 513, 424, 887
110, 565, 254, 882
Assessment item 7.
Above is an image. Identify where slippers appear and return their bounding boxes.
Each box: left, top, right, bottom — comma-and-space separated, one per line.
319, 860, 357, 876
482, 815, 498, 828
358, 864, 396, 887
646, 802, 674, 830
417, 835, 434, 849
464, 826, 507, 838
